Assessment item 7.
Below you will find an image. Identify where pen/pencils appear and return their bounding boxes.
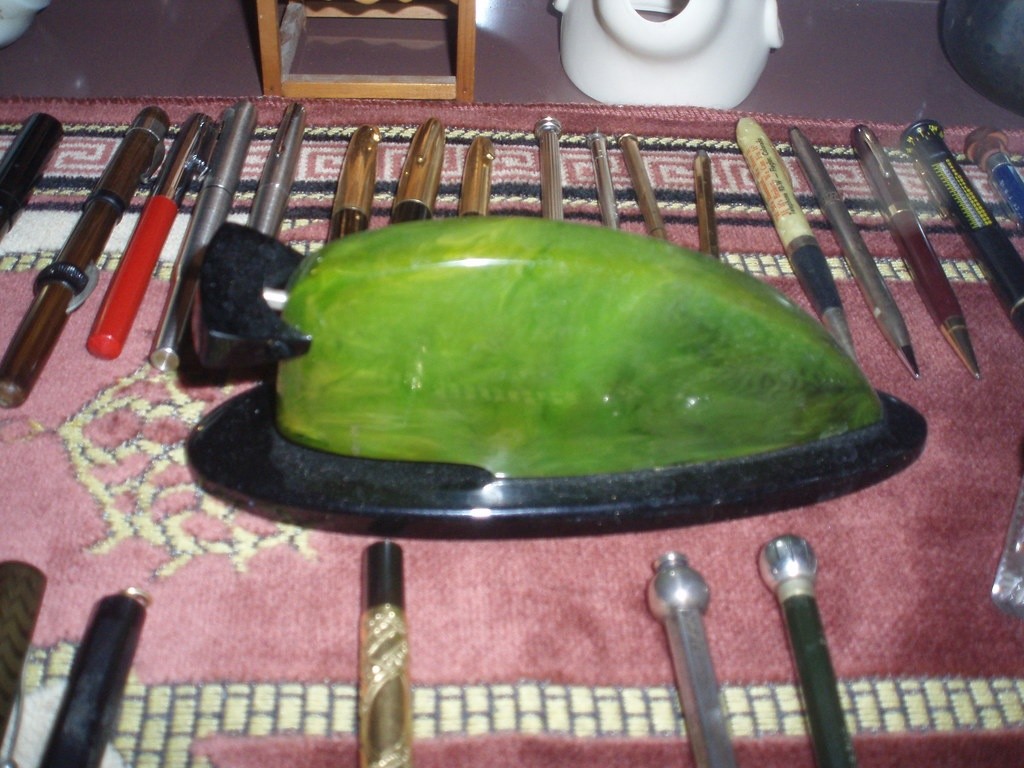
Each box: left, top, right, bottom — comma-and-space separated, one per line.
694, 148, 721, 257
38, 586, 150, 768
583, 128, 623, 235
756, 535, 853, 768
85, 114, 216, 362
356, 540, 417, 768
532, 116, 565, 218
645, 552, 742, 768
241, 103, 312, 240
0, 114, 62, 248
900, 122, 1023, 340
735, 116, 855, 362
789, 126, 919, 379
0, 103, 173, 413
147, 97, 262, 374
851, 121, 982, 382
0, 558, 48, 753
615, 133, 671, 239
322, 118, 493, 246
